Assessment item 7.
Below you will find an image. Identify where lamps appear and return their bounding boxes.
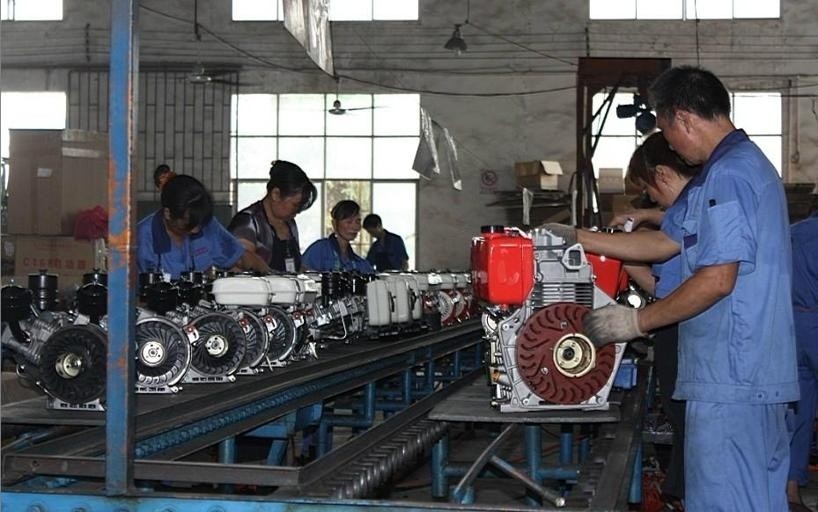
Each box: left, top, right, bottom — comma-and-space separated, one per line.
445, 0, 475, 59
326, 75, 348, 116
185, 0, 211, 85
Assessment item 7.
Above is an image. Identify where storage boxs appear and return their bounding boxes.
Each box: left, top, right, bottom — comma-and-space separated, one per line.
512, 160, 563, 189
596, 167, 639, 226
7, 127, 108, 288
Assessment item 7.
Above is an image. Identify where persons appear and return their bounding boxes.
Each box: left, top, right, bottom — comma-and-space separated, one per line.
224, 160, 318, 274
154, 164, 176, 187
304, 204, 374, 272
136, 174, 271, 282
363, 213, 414, 273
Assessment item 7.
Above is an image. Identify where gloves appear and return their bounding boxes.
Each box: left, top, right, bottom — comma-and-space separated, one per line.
538, 222, 579, 248
582, 304, 643, 346
